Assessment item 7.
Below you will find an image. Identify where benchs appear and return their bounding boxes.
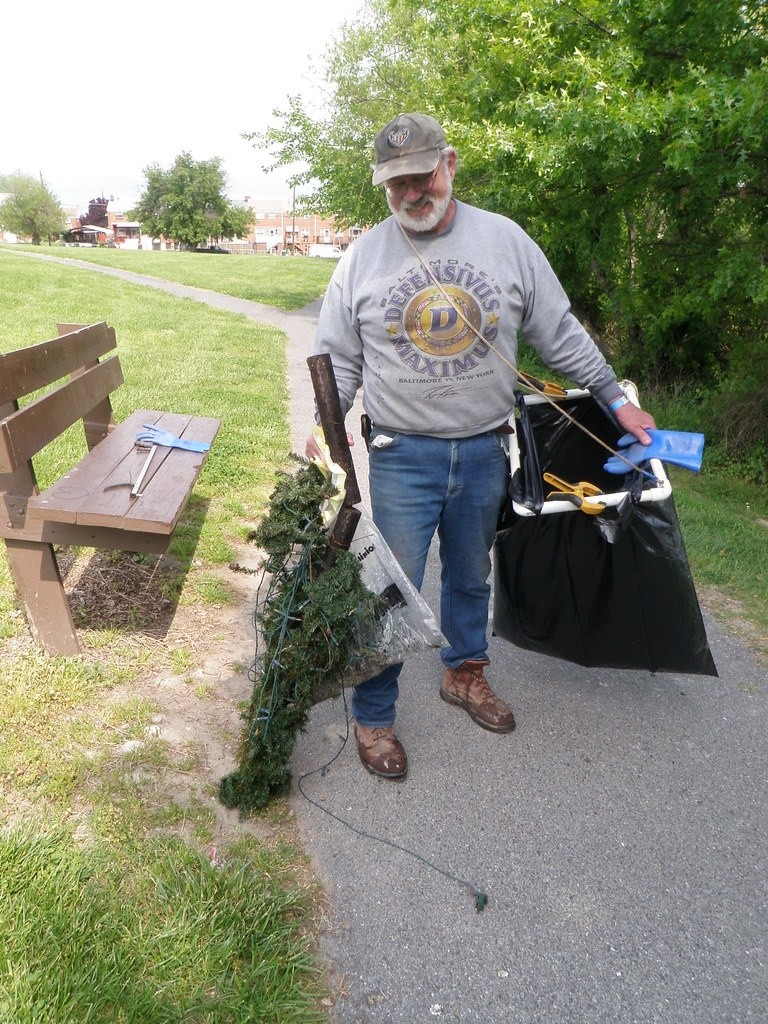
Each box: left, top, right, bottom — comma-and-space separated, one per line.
0, 322, 222, 658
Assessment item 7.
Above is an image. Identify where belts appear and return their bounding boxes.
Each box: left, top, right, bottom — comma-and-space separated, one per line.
494, 425, 514, 434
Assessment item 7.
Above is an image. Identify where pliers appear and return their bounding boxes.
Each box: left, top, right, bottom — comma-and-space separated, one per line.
543, 472, 607, 515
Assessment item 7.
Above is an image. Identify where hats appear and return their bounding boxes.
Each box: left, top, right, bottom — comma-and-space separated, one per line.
372, 113, 447, 186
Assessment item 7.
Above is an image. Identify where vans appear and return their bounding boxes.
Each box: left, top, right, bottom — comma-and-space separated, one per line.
308, 244, 345, 258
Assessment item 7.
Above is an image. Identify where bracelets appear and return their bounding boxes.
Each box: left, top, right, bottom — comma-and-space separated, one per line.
608, 395, 629, 414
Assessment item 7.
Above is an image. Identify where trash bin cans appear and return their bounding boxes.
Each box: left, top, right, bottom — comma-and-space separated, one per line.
282, 250, 287, 256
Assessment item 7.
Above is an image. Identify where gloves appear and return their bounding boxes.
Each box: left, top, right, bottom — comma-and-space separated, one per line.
603, 429, 706, 473
136, 424, 210, 452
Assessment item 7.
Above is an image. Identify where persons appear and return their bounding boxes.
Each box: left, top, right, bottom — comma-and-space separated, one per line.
304, 113, 659, 778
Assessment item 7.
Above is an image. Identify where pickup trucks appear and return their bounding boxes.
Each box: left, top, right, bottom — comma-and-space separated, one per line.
195, 245, 231, 254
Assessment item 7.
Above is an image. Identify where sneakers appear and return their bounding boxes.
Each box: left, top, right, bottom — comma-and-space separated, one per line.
440, 661, 516, 734
353, 721, 408, 778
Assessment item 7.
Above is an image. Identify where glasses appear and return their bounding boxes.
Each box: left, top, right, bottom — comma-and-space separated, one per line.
384, 157, 444, 197
